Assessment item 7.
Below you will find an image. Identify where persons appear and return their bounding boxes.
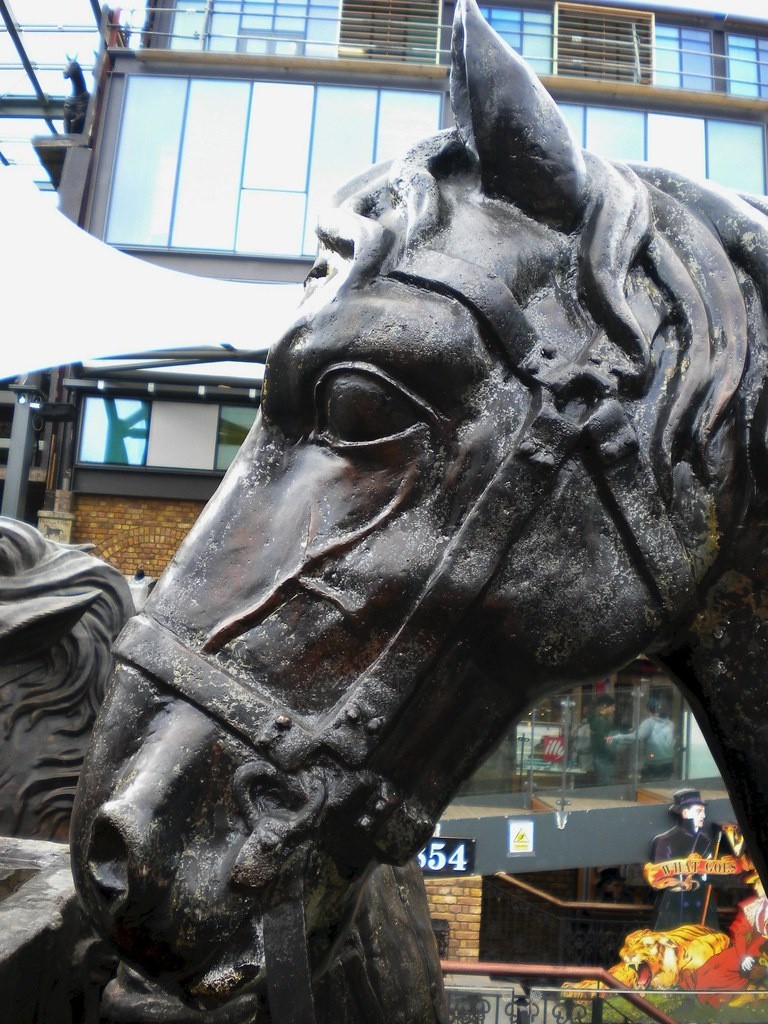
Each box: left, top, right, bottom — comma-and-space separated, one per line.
583, 705, 589, 717
595, 867, 635, 904
651, 787, 723, 932
590, 694, 676, 786
572, 718, 590, 770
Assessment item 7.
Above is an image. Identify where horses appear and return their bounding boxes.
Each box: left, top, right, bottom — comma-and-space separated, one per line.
0, 514, 449, 1024
69, 0, 767, 980
